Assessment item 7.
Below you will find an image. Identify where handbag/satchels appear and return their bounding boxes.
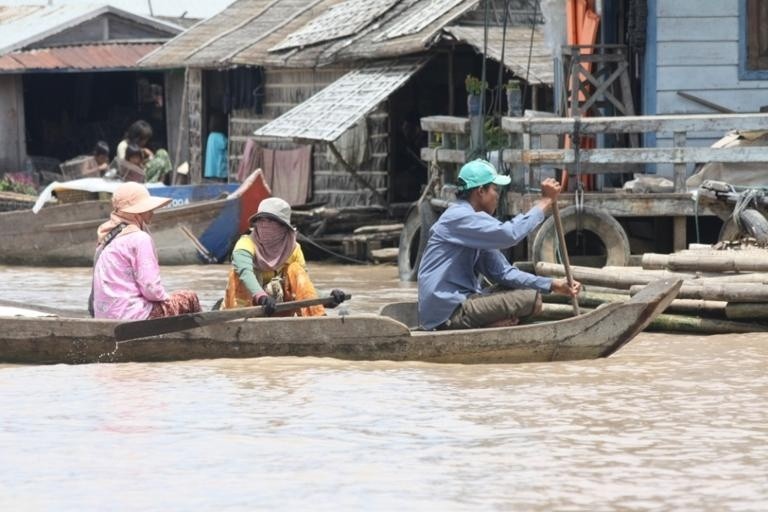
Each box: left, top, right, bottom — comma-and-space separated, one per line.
88, 290, 94, 316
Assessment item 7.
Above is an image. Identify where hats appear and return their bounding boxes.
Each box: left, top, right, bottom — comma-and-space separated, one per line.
247, 197, 297, 232
456, 158, 512, 191
112, 181, 173, 214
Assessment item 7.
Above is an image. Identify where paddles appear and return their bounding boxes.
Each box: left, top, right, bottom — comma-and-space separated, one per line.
114, 294, 351, 342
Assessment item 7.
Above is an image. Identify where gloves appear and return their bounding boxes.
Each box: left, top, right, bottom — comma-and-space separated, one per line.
323, 289, 346, 309
254, 291, 278, 316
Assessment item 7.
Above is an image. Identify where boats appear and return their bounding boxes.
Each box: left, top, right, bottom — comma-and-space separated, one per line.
0, 166, 272, 268
1, 271, 685, 371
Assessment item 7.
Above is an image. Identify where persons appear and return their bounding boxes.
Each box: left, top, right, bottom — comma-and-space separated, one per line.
88, 179, 203, 324
222, 195, 328, 322
114, 119, 173, 188
199, 112, 229, 184
80, 141, 112, 182
415, 156, 584, 331
